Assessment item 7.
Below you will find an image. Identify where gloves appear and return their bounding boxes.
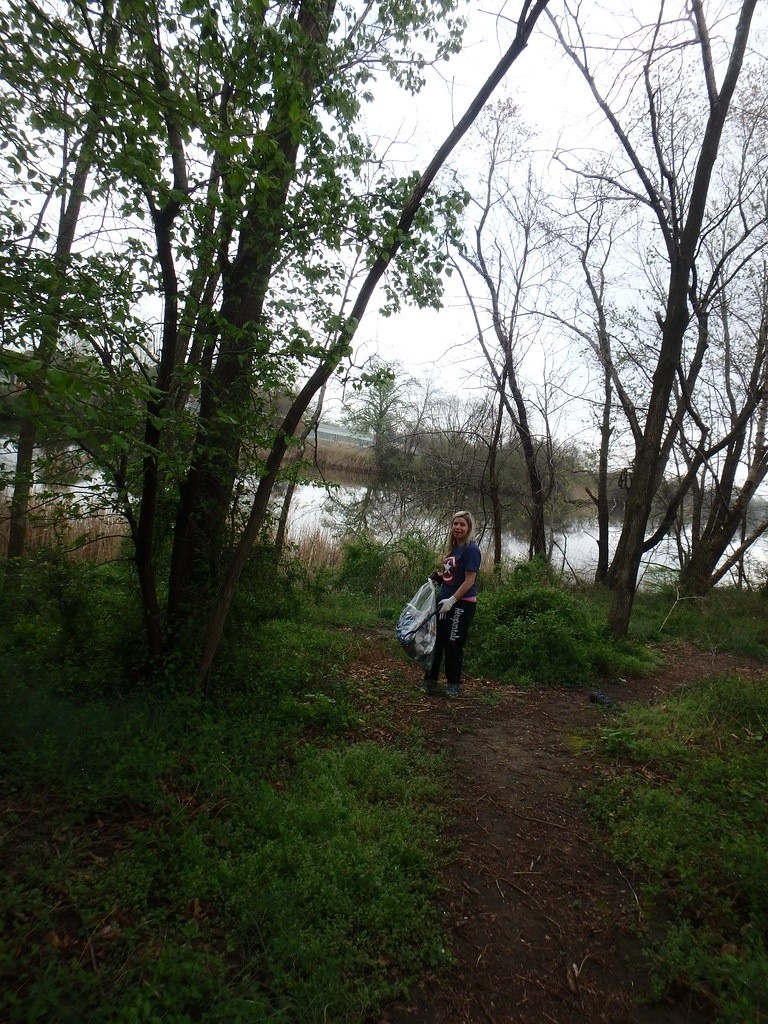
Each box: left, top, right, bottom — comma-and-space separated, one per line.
438, 596, 457, 615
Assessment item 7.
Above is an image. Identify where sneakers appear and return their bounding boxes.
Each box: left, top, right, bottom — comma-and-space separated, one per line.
422, 679, 460, 698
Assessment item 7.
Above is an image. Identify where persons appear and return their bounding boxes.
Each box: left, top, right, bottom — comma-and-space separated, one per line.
414, 509, 482, 698
